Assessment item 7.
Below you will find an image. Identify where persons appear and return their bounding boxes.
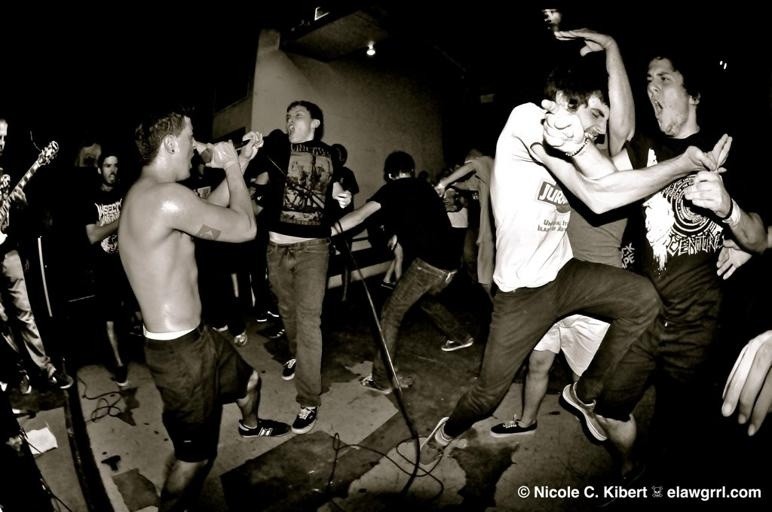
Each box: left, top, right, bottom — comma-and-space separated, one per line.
711, 207, 771, 448
117, 100, 292, 512
247, 100, 351, 436
0, 118, 73, 392
330, 149, 474, 396
543, 46, 772, 507
414, 60, 733, 467
488, 28, 646, 438
434, 148, 498, 300
80, 151, 144, 387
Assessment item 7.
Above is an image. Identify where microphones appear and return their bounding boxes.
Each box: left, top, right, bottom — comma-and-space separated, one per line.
201, 138, 260, 161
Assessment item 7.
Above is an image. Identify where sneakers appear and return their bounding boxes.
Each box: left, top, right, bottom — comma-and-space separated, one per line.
267, 308, 280, 318
562, 382, 609, 441
16, 372, 32, 394
418, 417, 454, 466
116, 364, 127, 386
239, 418, 291, 438
291, 406, 317, 434
598, 463, 643, 510
489, 419, 537, 438
282, 358, 297, 381
211, 323, 228, 333
358, 376, 393, 395
39, 372, 70, 390
441, 337, 474, 351
381, 280, 395, 290
256, 316, 268, 322
234, 328, 248, 347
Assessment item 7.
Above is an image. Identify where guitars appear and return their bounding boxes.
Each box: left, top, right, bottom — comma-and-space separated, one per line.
0, 141, 61, 245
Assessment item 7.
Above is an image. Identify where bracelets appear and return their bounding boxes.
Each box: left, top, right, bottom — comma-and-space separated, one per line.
721, 198, 733, 220
565, 137, 589, 157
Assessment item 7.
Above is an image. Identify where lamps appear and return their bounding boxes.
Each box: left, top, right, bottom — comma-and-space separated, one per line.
365, 40, 376, 55
